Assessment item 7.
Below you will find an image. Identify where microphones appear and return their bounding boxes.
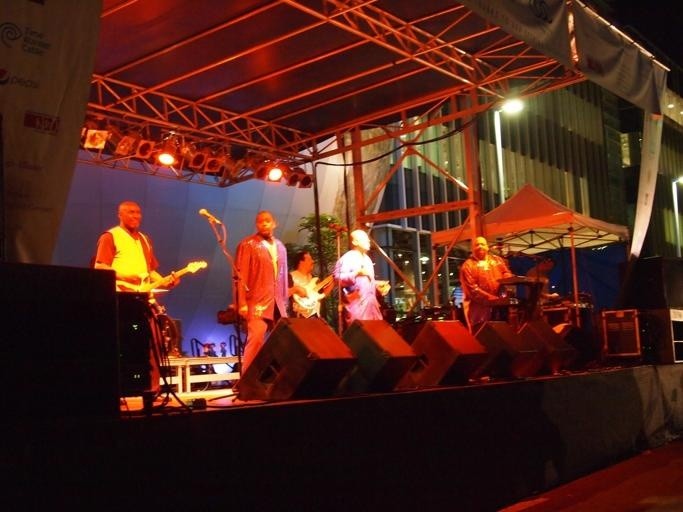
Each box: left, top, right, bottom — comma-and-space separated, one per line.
328, 223, 348, 232
199, 208, 223, 226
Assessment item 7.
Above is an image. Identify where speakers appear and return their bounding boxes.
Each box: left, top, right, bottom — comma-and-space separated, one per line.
334, 319, 417, 394
470, 321, 537, 381
0, 262, 121, 416
513, 315, 574, 372
234, 317, 358, 401
394, 319, 487, 391
618, 256, 682, 308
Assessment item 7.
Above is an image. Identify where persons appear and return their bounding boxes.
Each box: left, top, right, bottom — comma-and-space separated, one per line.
232, 211, 288, 375
458, 236, 509, 328
94, 200, 182, 406
523, 255, 559, 304
287, 252, 322, 320
333, 229, 388, 334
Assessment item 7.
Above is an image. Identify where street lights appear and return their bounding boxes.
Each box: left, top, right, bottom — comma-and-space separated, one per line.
492, 98, 524, 205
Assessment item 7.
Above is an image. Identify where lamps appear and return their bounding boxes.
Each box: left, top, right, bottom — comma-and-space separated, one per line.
80, 112, 314, 189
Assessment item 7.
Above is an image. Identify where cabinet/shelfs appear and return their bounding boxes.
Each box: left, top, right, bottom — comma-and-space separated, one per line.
647, 308, 683, 364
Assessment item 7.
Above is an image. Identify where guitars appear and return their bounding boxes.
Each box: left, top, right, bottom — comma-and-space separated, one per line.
116, 261, 208, 303
293, 273, 333, 308
